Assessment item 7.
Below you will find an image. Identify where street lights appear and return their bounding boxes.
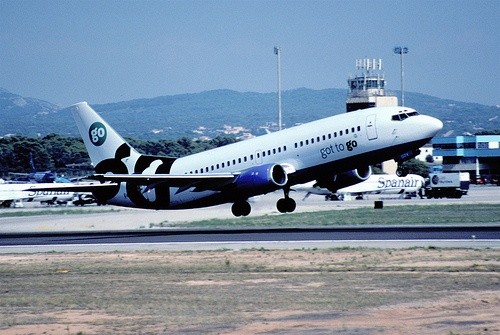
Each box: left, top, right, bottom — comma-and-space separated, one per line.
394, 46, 408, 105
274, 45, 282, 130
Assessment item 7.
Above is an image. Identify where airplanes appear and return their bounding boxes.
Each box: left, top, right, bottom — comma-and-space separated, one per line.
0, 101, 444, 217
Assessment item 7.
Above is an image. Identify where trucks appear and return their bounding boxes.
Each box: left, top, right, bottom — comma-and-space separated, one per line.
418, 173, 470, 198
